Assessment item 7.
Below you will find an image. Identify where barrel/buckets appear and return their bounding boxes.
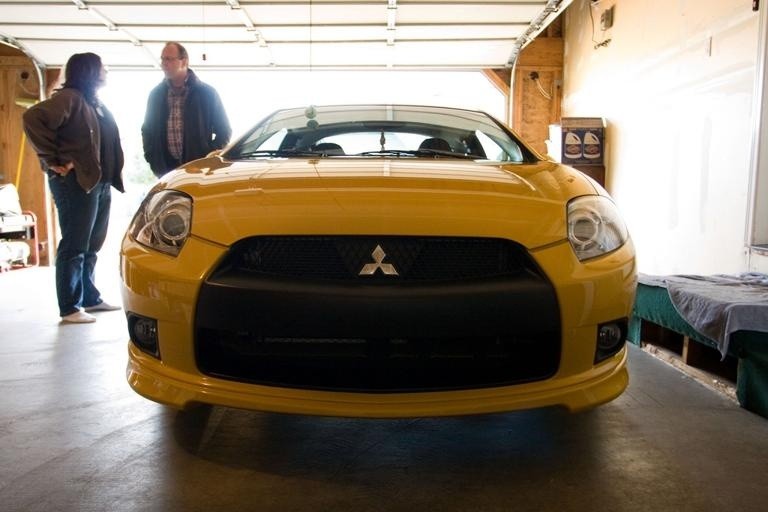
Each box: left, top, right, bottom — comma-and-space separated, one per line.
582, 129, 601, 159
563, 128, 582, 160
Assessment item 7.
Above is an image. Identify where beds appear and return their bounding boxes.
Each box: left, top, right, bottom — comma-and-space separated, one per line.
626, 272, 768, 418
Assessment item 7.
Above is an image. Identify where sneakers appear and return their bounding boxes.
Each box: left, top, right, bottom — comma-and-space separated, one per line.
63, 308, 96, 323
84, 301, 121, 311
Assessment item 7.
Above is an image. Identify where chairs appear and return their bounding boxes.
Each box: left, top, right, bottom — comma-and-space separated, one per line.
0, 184, 40, 269
312, 143, 345, 154
419, 138, 451, 156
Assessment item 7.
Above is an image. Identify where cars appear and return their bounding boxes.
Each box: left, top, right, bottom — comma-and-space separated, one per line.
118, 104, 637, 421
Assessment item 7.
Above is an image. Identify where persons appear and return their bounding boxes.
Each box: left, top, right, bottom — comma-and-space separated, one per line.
22, 53, 127, 324
140, 42, 232, 180
